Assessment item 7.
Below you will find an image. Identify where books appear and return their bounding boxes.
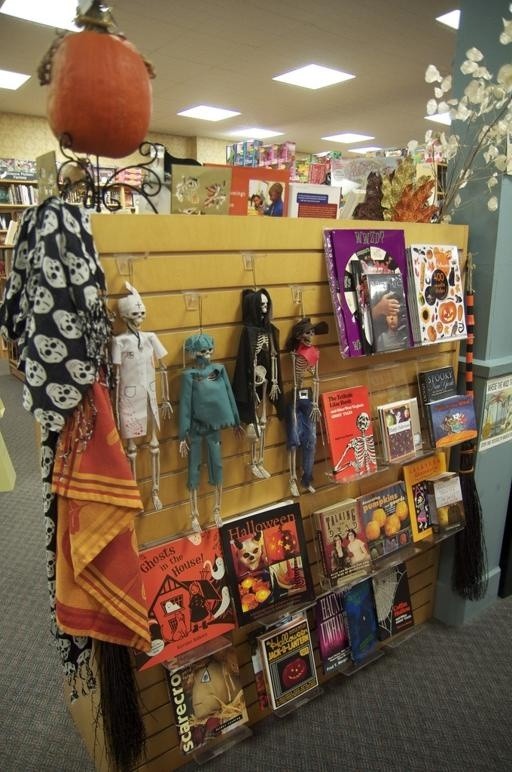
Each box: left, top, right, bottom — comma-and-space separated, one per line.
1, 181, 121, 242
165, 644, 249, 757
402, 450, 447, 543
312, 498, 373, 589
321, 384, 377, 481
424, 472, 466, 535
219, 499, 316, 629
253, 561, 417, 709
321, 232, 471, 360
419, 364, 478, 452
139, 527, 235, 674
355, 480, 413, 561
375, 397, 423, 465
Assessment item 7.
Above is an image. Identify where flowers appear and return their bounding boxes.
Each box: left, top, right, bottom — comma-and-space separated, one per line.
423, 5, 511, 223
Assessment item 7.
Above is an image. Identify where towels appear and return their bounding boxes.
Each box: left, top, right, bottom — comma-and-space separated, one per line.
50, 371, 152, 653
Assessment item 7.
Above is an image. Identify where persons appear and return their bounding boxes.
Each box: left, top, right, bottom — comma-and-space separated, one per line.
254, 195, 262, 209
265, 183, 283, 217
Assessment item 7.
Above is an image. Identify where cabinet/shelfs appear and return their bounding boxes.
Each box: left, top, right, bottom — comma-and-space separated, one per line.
0, 178, 137, 358
